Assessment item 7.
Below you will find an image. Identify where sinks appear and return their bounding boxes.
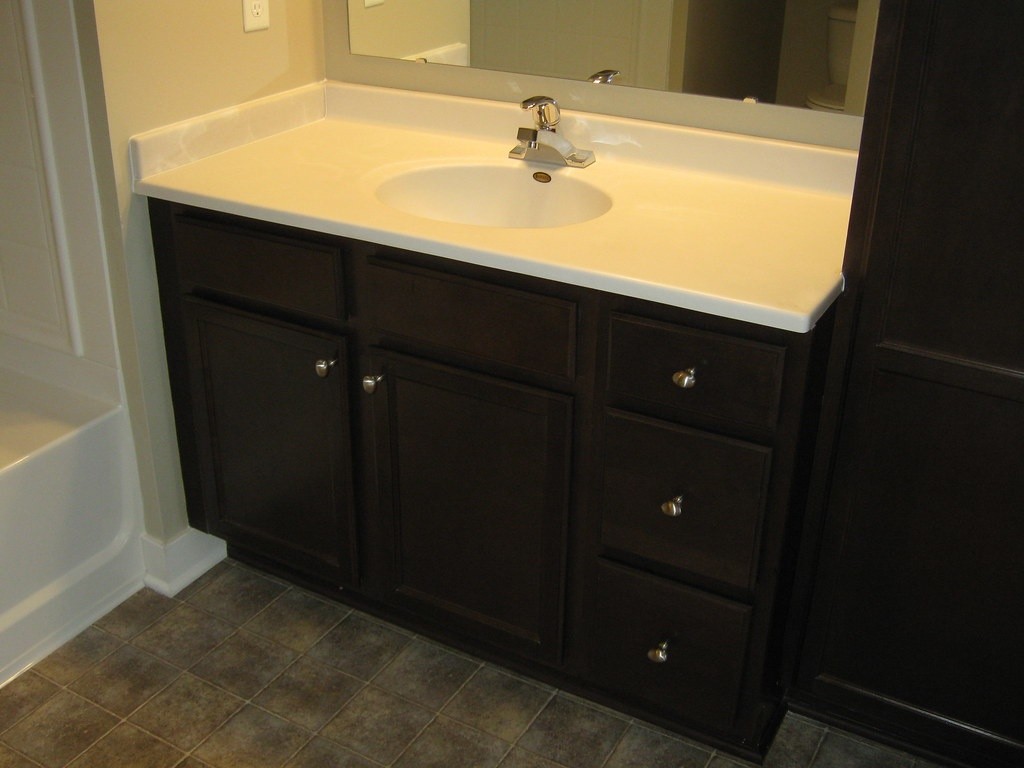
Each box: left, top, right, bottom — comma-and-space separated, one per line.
375, 164, 613, 230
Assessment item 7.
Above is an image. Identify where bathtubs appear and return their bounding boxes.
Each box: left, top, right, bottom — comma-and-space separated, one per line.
0, 366, 146, 689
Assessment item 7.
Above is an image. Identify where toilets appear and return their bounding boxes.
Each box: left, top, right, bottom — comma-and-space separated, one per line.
805, 6, 858, 114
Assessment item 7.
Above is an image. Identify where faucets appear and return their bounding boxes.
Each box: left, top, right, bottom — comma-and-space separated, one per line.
507, 95, 596, 169
